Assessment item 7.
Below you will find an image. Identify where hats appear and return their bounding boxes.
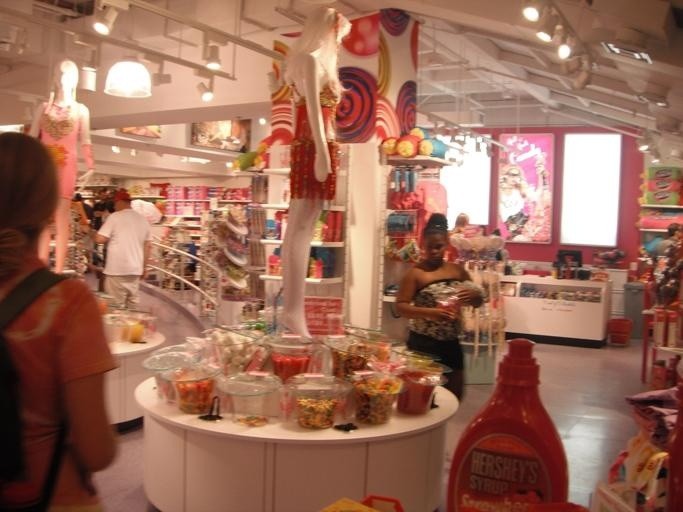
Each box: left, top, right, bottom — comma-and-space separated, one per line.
112, 188, 131, 202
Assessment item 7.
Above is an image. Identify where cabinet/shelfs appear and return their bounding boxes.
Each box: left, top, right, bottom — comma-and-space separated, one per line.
593, 344, 683, 512
498, 273, 612, 341
606, 269, 627, 314
376, 155, 452, 305
105, 331, 170, 424
132, 377, 460, 512
159, 198, 252, 282
638, 203, 683, 233
258, 166, 351, 318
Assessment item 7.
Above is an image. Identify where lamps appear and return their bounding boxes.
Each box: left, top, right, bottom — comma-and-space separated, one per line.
521, 1, 550, 22
92, 6, 117, 37
151, 70, 174, 87
557, 32, 572, 59
197, 81, 215, 101
78, 66, 96, 94
203, 42, 222, 73
536, 15, 561, 44
102, 55, 153, 100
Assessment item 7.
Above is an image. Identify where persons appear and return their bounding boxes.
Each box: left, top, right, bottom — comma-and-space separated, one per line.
29, 58, 96, 276
97, 191, 152, 312
498, 162, 543, 240
72, 194, 94, 226
654, 224, 683, 263
0, 131, 118, 512
453, 213, 470, 234
94, 190, 111, 230
280, 5, 353, 337
394, 213, 485, 403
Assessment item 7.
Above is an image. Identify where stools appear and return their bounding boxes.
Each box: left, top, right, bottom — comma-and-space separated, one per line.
641, 306, 679, 384
